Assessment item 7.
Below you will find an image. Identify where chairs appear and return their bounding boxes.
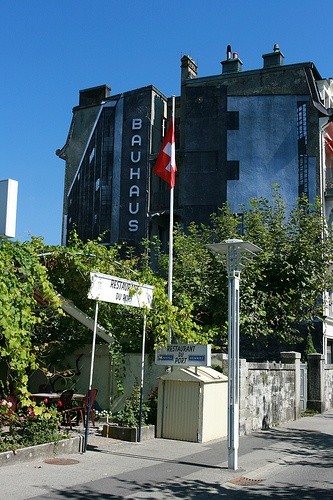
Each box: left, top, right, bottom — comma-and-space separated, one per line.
55, 389, 98, 429
39, 384, 52, 405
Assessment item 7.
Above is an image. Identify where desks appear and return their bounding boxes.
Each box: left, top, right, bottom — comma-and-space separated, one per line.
30, 393, 85, 406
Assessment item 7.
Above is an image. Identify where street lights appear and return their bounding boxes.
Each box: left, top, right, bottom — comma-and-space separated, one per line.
204, 239, 263, 472
